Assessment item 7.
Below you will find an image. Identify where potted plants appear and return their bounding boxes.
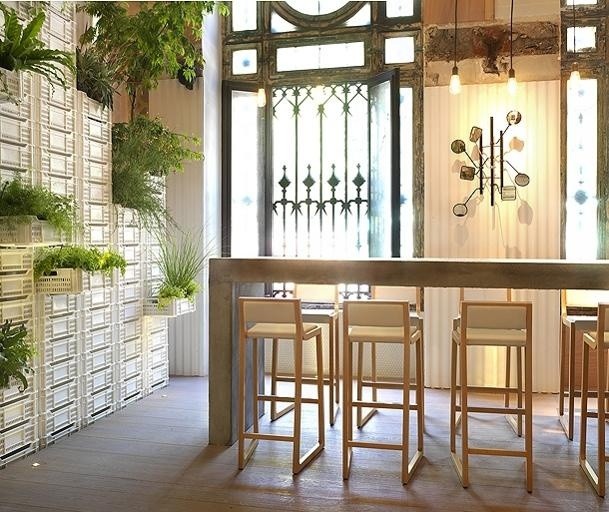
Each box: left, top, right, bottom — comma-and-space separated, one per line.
33, 243, 127, 295
0, 169, 85, 247
143, 228, 217, 318
0, 319, 36, 393
75, 1, 232, 110
0, 1, 77, 106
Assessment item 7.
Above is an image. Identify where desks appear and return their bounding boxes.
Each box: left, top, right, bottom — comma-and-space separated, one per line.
209, 258, 609, 446
